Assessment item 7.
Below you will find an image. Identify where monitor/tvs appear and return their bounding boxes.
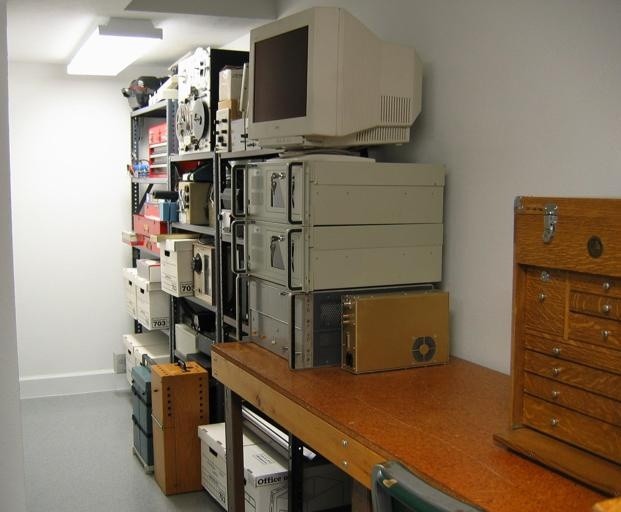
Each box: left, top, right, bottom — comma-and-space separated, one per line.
247, 5, 425, 157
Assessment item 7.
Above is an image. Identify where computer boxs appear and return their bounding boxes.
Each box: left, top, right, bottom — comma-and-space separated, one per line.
230, 225, 445, 291
230, 158, 447, 223
233, 276, 431, 371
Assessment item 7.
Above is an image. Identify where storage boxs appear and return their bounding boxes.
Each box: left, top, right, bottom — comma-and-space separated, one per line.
490, 197, 621, 496
120, 68, 242, 388
195, 422, 350, 512
150, 361, 209, 497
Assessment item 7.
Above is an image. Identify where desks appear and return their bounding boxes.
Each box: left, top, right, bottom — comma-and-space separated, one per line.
208, 340, 621, 512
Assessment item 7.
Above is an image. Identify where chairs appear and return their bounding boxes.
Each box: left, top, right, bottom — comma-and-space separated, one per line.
371, 459, 479, 511
